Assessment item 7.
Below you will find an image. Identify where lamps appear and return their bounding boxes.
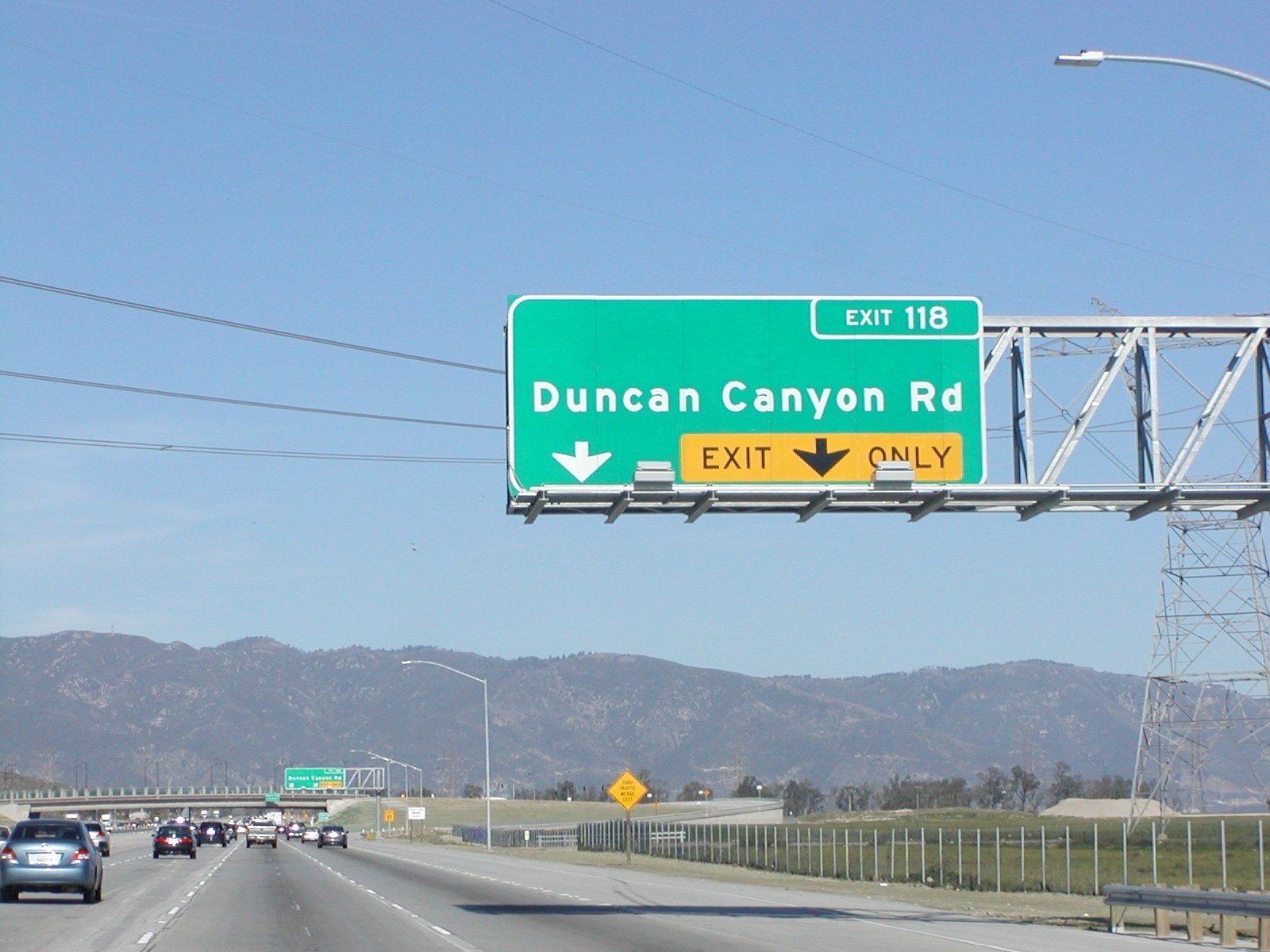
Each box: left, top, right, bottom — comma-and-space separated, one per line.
871, 462, 914, 489
632, 459, 676, 493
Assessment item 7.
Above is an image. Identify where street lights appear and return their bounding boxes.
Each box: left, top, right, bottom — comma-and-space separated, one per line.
371, 756, 409, 838
400, 660, 492, 850
348, 749, 392, 839
390, 761, 424, 836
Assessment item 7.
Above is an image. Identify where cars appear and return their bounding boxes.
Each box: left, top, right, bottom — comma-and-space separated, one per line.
75, 822, 112, 857
166, 817, 320, 849
317, 825, 349, 849
0, 824, 12, 854
151, 824, 200, 859
0, 818, 103, 904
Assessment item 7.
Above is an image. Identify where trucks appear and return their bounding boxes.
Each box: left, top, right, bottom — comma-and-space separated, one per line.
100, 814, 112, 827
65, 813, 82, 820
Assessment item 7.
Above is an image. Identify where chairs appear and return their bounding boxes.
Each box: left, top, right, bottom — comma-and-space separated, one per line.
62, 830, 77, 839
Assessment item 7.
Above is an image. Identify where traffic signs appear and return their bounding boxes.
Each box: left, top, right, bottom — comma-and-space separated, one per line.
284, 767, 346, 791
504, 293, 987, 501
